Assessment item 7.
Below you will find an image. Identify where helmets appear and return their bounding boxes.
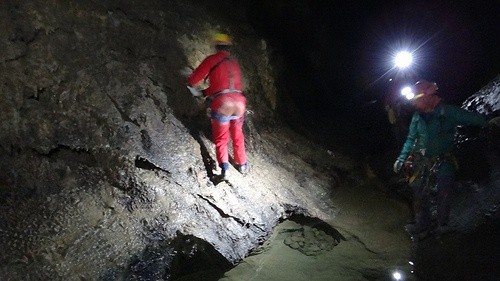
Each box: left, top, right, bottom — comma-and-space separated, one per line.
211, 33, 234, 47
410, 80, 439, 102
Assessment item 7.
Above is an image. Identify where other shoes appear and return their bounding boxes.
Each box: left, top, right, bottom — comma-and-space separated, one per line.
220, 169, 231, 181
238, 162, 251, 174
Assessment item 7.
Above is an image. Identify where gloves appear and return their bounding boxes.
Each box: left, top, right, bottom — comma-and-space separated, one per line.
392, 159, 404, 174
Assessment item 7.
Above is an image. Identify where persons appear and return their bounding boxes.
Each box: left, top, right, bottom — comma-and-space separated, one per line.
187, 32, 250, 180
394, 79, 500, 234
